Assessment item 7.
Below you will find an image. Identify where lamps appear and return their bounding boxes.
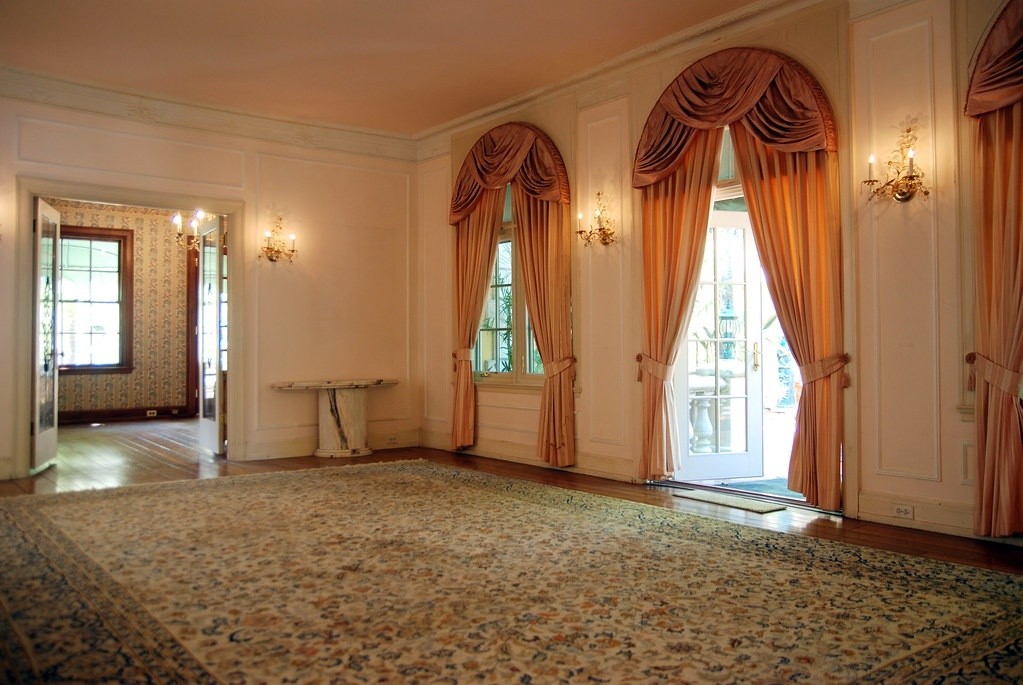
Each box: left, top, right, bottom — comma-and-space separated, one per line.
257, 216, 299, 267
718, 297, 740, 361
575, 205, 616, 249
172, 210, 219, 252
862, 146, 935, 207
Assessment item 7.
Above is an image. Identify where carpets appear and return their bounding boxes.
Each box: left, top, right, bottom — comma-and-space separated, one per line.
717, 476, 803, 499
0, 456, 1023, 685
673, 488, 788, 515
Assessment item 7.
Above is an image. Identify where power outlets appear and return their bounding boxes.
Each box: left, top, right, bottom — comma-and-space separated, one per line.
146, 410, 158, 417
894, 504, 914, 520
387, 436, 400, 443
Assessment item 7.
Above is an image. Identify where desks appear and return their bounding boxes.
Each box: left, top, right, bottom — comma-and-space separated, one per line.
688, 373, 726, 451
